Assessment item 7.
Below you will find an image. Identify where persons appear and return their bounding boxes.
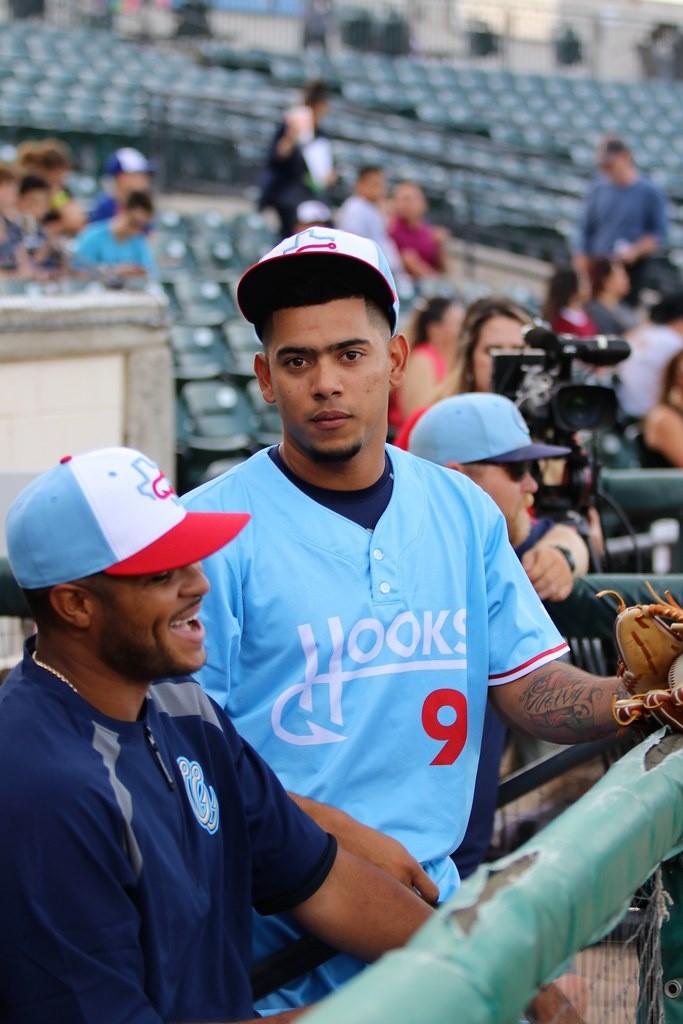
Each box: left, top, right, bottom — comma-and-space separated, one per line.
261, 83, 683, 816
177, 225, 682, 1019
408, 394, 588, 1024
0, 142, 162, 303
0, 447, 437, 1024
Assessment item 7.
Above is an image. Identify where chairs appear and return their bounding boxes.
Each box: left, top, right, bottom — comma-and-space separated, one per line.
0, 20, 681, 493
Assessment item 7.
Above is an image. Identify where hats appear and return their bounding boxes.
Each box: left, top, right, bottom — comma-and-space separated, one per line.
296, 198, 334, 224
235, 227, 401, 339
408, 392, 573, 466
4, 446, 252, 589
107, 146, 157, 174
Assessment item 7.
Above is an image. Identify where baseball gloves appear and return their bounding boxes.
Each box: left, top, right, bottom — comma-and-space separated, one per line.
594, 579, 683, 737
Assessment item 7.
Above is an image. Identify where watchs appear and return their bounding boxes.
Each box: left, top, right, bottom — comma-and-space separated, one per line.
551, 544, 575, 573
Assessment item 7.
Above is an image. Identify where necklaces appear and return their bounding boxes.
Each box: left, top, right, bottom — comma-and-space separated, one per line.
29, 650, 78, 693
